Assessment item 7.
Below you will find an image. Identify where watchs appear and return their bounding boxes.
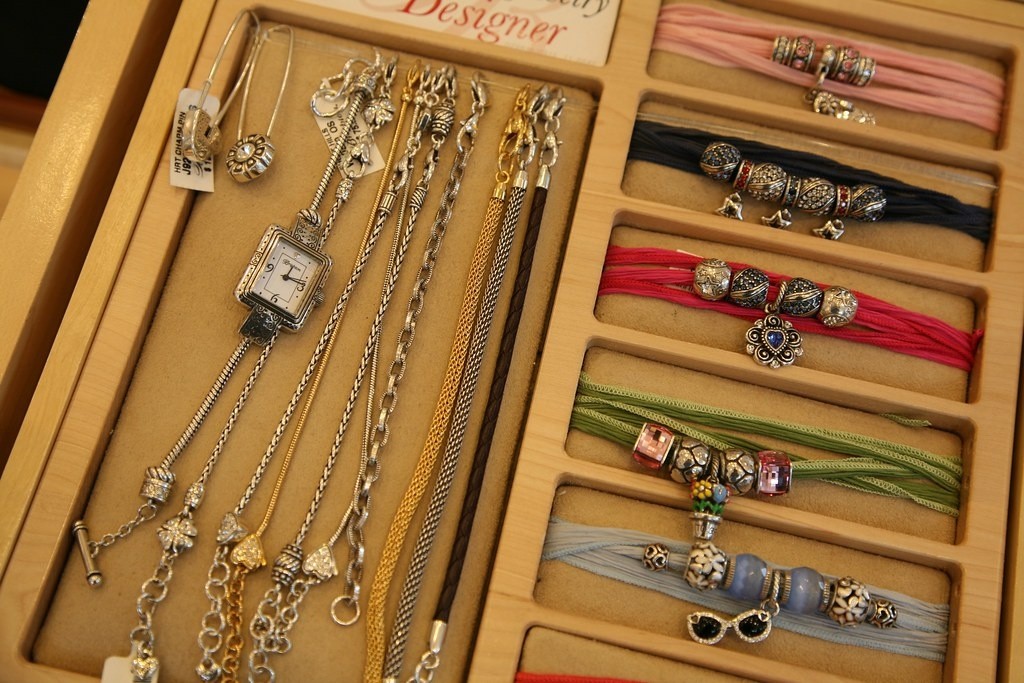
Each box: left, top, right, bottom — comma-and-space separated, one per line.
75, 49, 391, 586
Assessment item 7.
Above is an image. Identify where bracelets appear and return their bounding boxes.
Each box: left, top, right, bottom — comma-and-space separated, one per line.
134, 60, 567, 682
649, 4, 1004, 141
599, 242, 982, 397
630, 111, 999, 268
544, 512, 951, 664
570, 377, 967, 540
